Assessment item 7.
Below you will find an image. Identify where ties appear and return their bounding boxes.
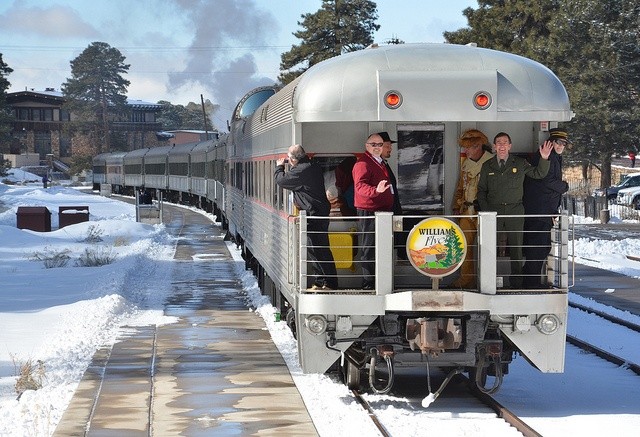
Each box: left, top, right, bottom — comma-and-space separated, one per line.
499, 158, 505, 171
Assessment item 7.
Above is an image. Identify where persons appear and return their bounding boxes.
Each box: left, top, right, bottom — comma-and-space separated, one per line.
523, 125, 572, 291
456, 136, 494, 288
289, 204, 294, 205
273, 143, 333, 294
477, 131, 554, 289
376, 129, 406, 216
42, 173, 49, 189
351, 132, 395, 294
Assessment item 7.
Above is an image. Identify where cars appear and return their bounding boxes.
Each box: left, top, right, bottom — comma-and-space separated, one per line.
617, 186, 640, 209
601, 173, 640, 205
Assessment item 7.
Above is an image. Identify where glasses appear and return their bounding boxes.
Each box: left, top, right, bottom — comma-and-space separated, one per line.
366, 141, 383, 146
554, 139, 567, 146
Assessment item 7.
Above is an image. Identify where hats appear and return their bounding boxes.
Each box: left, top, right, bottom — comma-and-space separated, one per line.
460, 129, 488, 146
377, 131, 398, 143
549, 127, 573, 144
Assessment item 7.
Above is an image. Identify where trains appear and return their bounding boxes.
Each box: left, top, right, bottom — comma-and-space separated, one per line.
93, 40, 576, 410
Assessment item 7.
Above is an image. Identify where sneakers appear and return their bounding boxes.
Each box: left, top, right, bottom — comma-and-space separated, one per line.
320, 285, 336, 292
308, 284, 321, 290
